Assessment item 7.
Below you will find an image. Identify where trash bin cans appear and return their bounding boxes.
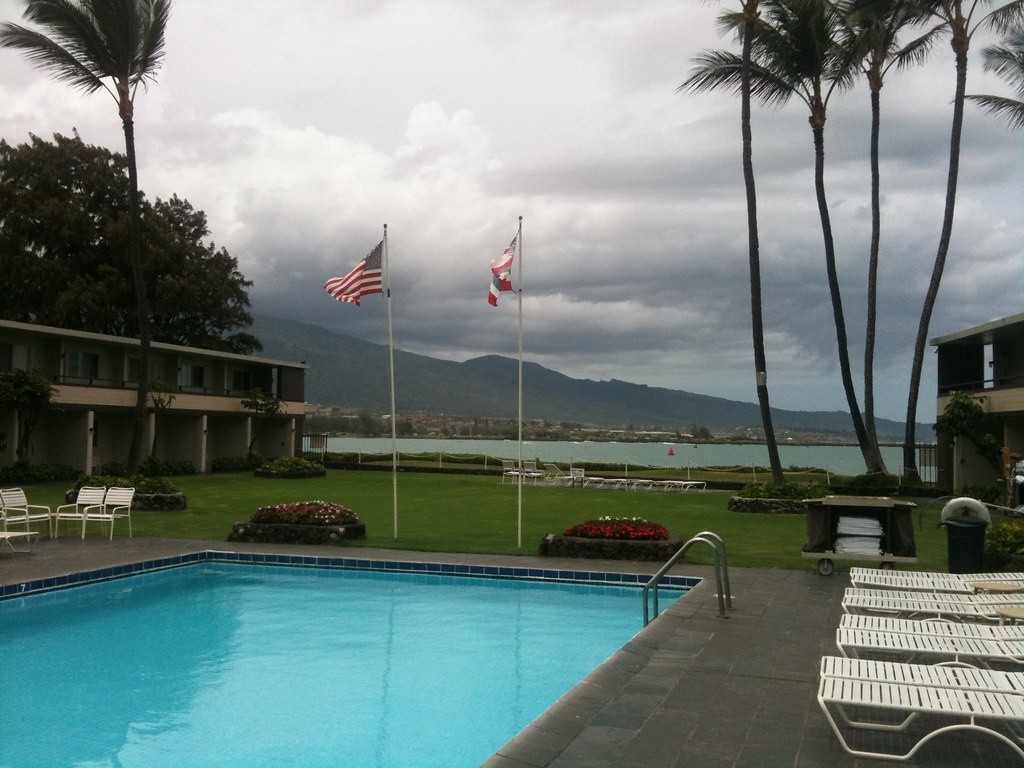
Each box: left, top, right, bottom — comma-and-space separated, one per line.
941, 496, 992, 574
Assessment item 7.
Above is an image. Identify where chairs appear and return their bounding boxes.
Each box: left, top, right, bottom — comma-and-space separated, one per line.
841, 587, 1024, 623
850, 567, 1024, 593
836, 614, 1024, 683
0, 488, 53, 546
817, 656, 1024, 761
0, 532, 40, 558
55, 486, 106, 540
83, 487, 136, 541
544, 460, 706, 494
502, 460, 545, 486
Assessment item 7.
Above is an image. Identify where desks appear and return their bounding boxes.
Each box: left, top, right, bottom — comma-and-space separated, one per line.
971, 582, 1022, 595
996, 608, 1024, 626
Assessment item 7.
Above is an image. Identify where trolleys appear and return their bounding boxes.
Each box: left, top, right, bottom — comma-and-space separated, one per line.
801, 495, 917, 576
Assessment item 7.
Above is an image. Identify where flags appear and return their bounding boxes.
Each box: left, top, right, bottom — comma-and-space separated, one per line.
488, 227, 523, 307
322, 234, 393, 305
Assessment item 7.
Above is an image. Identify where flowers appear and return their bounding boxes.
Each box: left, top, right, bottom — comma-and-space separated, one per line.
565, 515, 670, 540
250, 500, 360, 525
